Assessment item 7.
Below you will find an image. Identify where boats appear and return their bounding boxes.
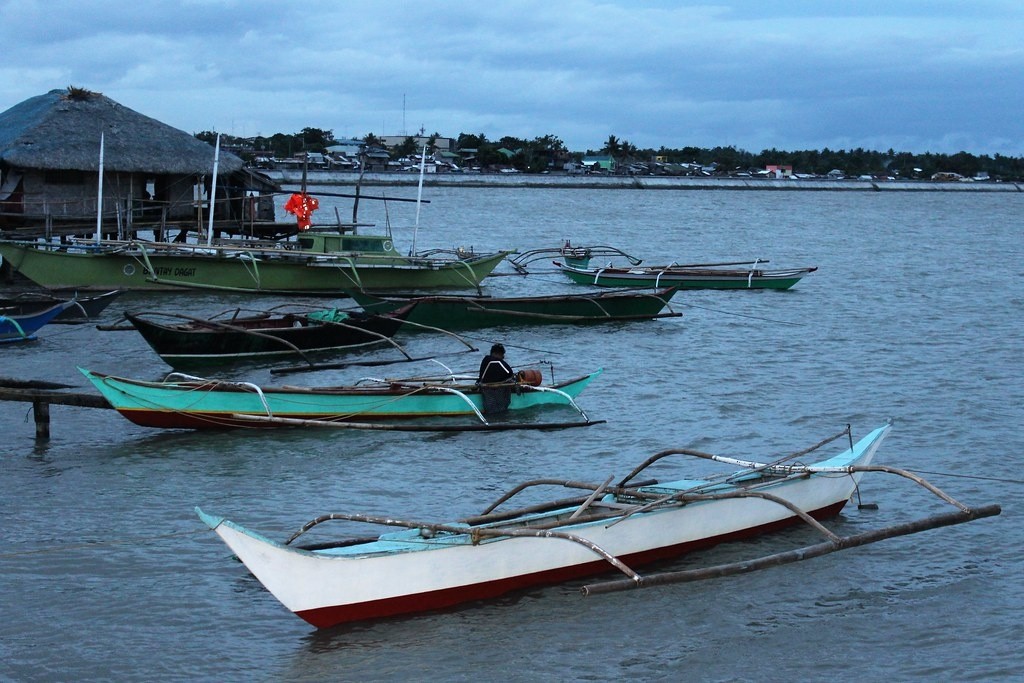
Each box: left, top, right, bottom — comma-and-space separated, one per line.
191, 419, 898, 631
552, 248, 819, 290
0, 231, 523, 296
95, 296, 420, 370
73, 363, 604, 430
0, 291, 91, 347
344, 284, 683, 326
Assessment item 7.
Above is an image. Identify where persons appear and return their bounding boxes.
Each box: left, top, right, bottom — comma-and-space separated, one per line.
480, 343, 512, 382
